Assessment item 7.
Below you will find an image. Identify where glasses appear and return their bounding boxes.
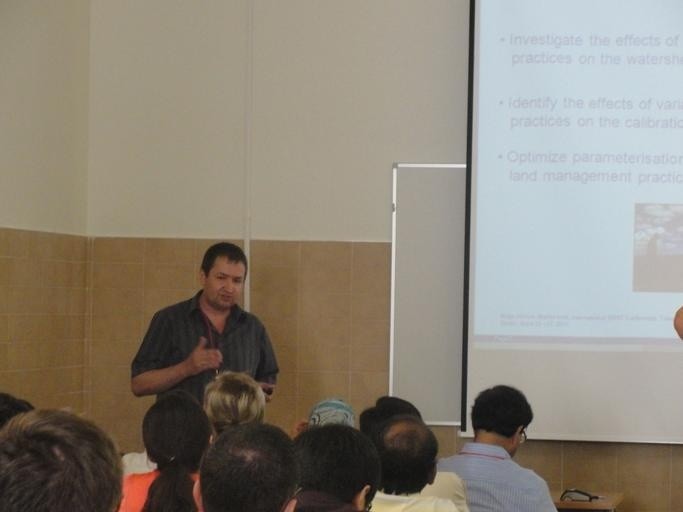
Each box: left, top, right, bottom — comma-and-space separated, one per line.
519, 430, 527, 445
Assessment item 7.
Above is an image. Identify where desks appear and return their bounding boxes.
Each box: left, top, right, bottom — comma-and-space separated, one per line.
549, 488, 626, 512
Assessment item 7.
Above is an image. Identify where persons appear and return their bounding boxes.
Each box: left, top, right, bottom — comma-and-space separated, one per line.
2, 372, 556, 512
130, 240, 279, 426
